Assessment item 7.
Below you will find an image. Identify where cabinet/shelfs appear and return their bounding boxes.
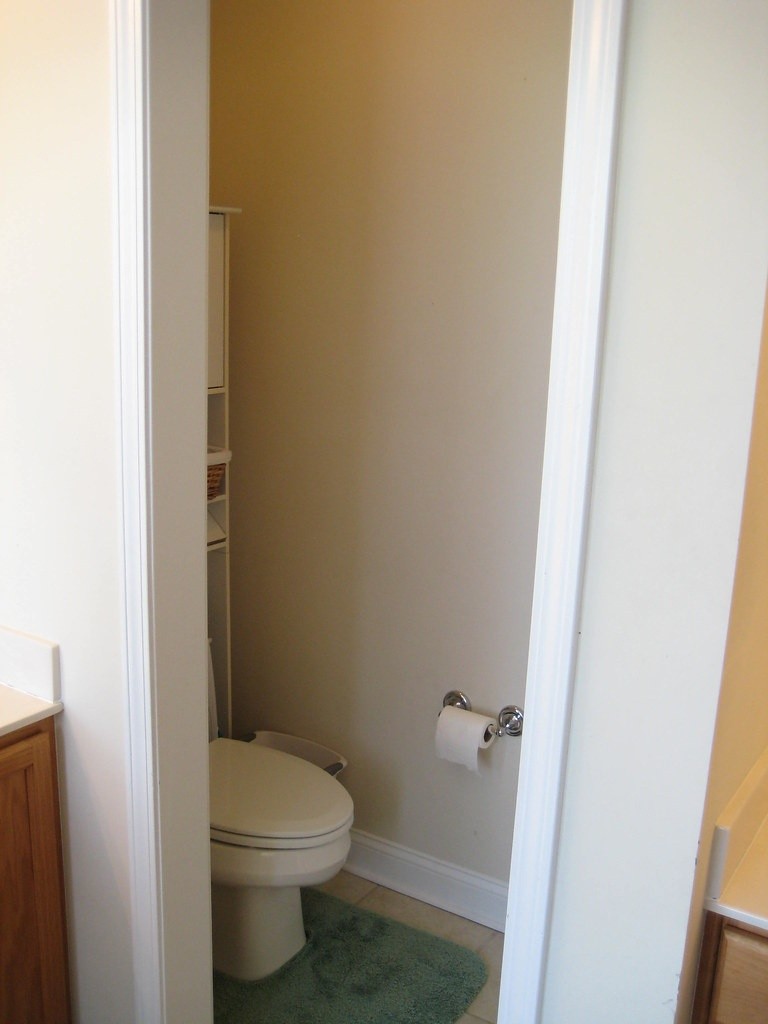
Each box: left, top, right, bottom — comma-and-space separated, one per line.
0, 715, 73, 1024
206, 206, 243, 743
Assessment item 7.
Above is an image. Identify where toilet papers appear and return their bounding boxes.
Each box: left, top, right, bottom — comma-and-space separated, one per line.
433, 704, 498, 770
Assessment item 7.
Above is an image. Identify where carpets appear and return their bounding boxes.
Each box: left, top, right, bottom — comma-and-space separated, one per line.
212, 885, 488, 1024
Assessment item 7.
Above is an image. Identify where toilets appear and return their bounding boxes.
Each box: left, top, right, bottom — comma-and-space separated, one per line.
207, 734, 356, 984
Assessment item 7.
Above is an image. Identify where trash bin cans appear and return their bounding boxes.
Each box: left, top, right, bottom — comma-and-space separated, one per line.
234, 729, 349, 779
